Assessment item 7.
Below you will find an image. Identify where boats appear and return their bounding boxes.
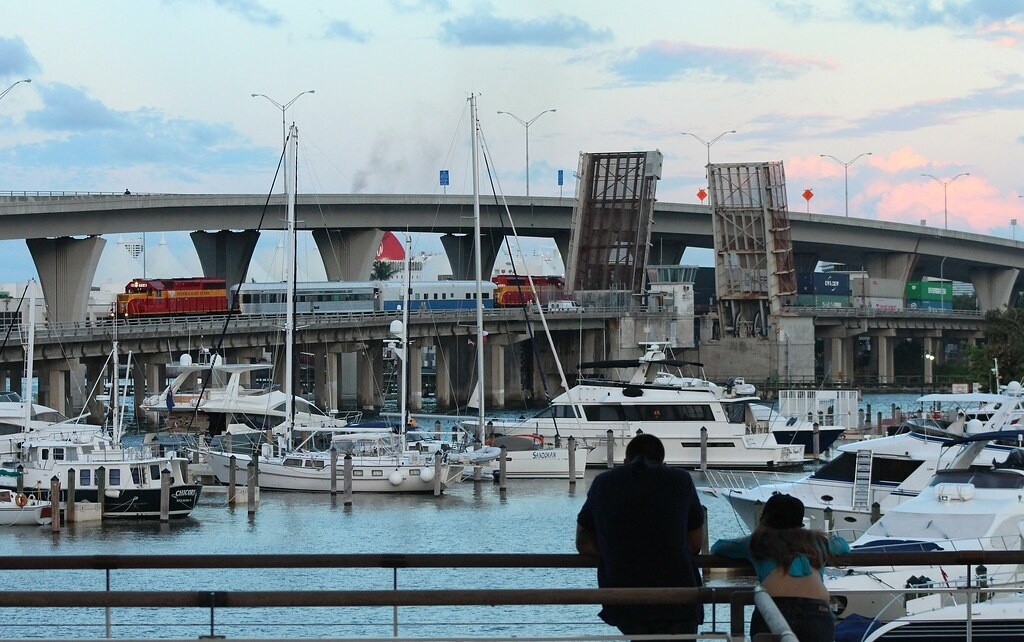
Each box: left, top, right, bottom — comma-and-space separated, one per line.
0, 90, 1024, 642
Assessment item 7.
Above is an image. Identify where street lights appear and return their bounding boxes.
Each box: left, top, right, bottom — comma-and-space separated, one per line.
922, 172, 970, 230
681, 130, 736, 206
497, 109, 557, 197
819, 152, 872, 218
250, 89, 316, 194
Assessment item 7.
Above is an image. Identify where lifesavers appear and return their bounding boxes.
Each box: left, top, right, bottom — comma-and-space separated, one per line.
28, 494, 36, 503
15, 494, 28, 507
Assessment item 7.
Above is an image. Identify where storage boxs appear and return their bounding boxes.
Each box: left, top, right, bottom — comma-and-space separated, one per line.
794, 270, 954, 314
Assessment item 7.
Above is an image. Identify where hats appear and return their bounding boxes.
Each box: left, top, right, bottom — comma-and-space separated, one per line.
759, 494, 805, 530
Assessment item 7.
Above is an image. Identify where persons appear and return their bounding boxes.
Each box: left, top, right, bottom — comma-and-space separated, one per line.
575, 434, 705, 642
713, 494, 849, 642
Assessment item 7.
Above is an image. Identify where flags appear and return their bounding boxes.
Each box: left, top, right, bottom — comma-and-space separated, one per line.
167, 387, 175, 412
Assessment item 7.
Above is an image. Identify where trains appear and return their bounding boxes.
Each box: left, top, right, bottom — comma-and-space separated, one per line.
111, 274, 569, 325
